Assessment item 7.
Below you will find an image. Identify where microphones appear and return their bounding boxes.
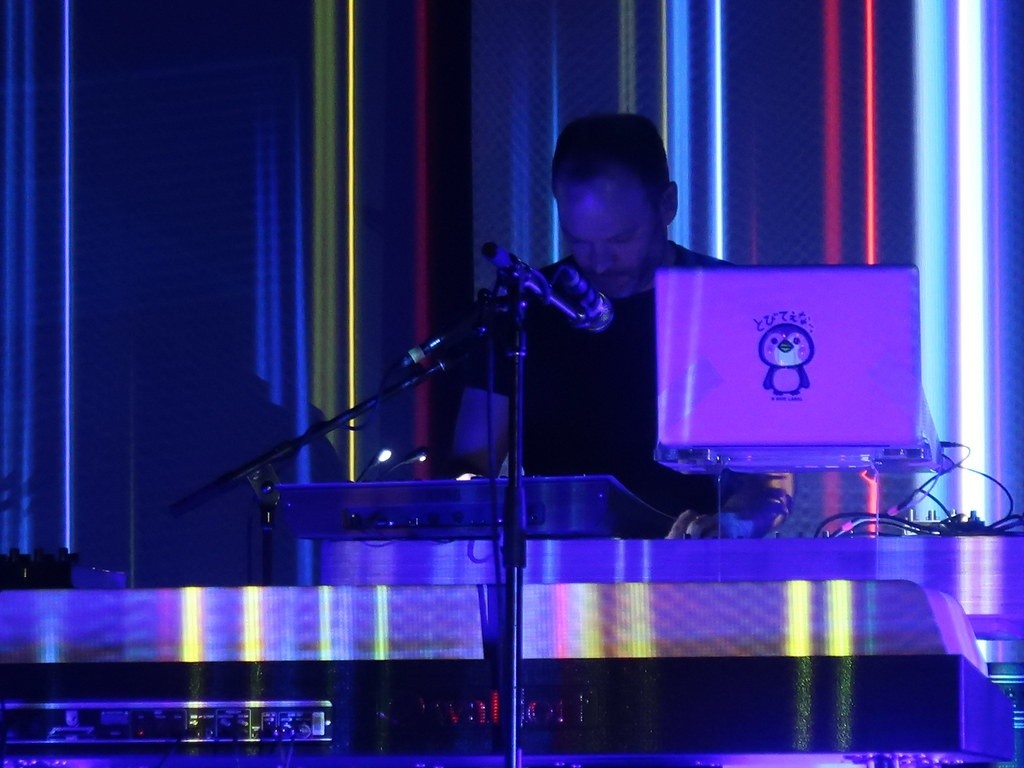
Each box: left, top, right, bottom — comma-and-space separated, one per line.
558, 267, 613, 332
398, 300, 502, 369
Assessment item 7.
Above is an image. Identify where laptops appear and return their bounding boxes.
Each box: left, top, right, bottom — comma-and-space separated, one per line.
652, 262, 931, 451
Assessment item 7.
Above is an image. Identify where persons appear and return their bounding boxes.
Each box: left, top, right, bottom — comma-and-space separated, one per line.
450, 112, 794, 539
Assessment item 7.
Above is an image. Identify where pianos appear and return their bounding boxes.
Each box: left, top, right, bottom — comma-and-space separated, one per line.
289, 534, 1024, 639
